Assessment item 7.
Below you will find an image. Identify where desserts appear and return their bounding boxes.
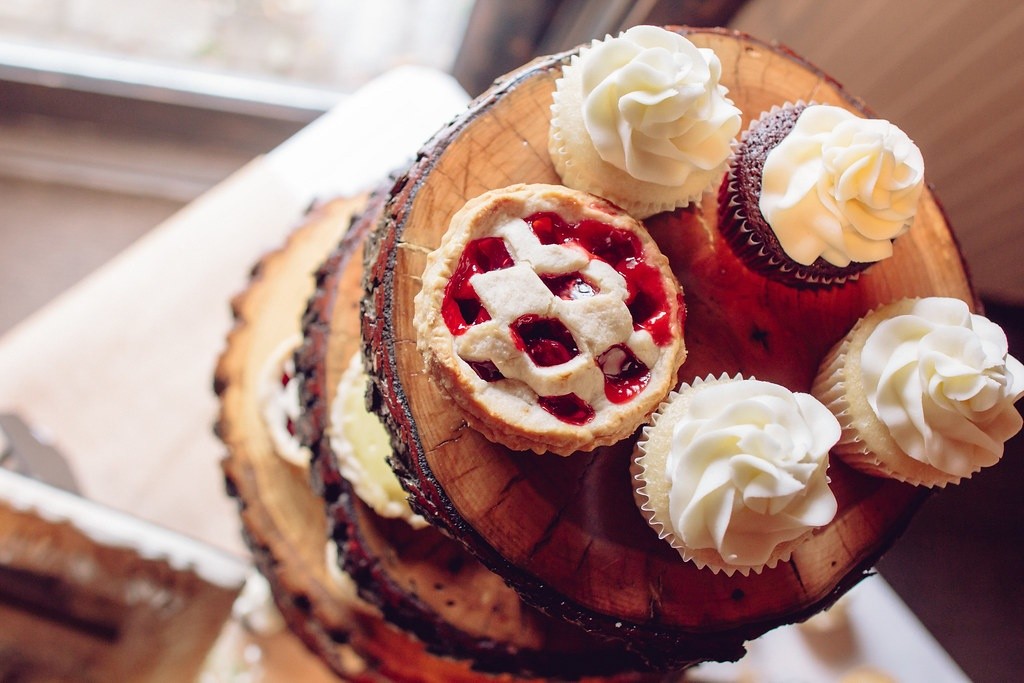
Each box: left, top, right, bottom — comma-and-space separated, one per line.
414, 24, 1024, 577
265, 339, 433, 616
